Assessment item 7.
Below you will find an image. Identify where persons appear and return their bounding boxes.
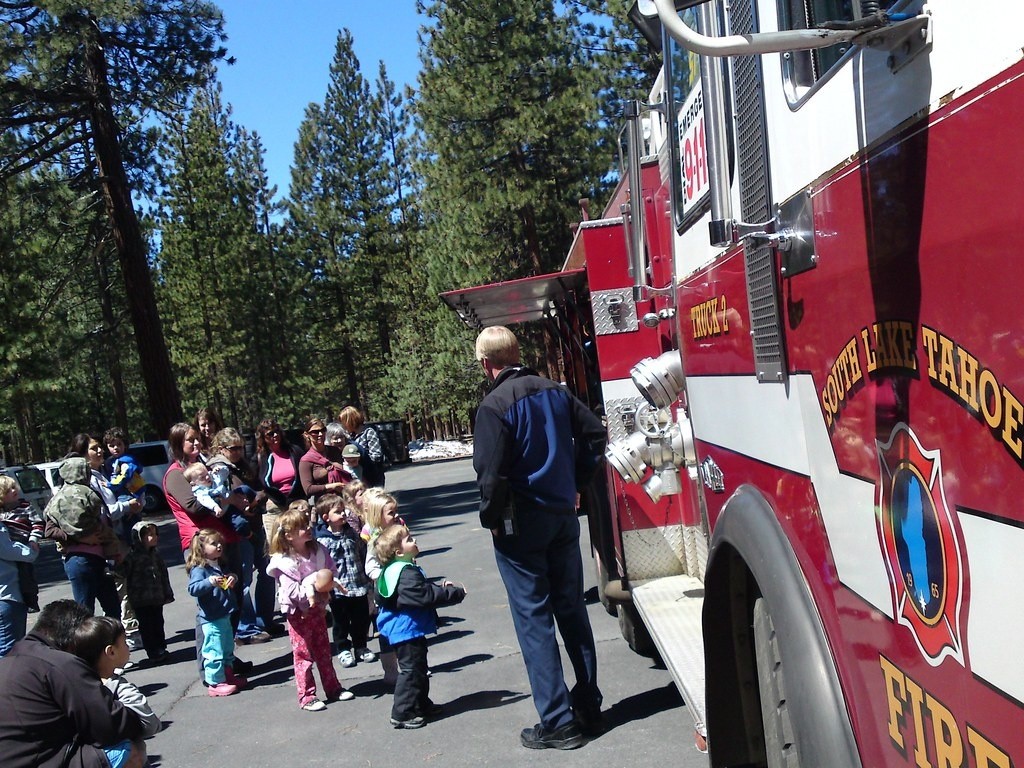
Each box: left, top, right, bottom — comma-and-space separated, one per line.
373, 525, 467, 729
188, 528, 247, 698
0, 406, 431, 768
266, 509, 354, 711
123, 520, 180, 665
472, 326, 608, 751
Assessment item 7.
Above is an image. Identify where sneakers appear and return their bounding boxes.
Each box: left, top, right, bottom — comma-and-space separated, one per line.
390, 716, 426, 730
415, 704, 444, 717
572, 701, 607, 736
328, 690, 354, 700
302, 699, 327, 710
126, 632, 144, 651
208, 683, 237, 697
355, 647, 378, 663
338, 650, 356, 667
521, 719, 582, 749
224, 666, 247, 689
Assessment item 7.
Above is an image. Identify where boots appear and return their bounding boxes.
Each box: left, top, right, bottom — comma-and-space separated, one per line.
379, 652, 400, 685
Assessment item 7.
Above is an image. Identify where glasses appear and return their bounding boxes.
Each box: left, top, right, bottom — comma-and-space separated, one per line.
263, 429, 280, 437
308, 427, 327, 434
226, 446, 243, 451
185, 437, 198, 443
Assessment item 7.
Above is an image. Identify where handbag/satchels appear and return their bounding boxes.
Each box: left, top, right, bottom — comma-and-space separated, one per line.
365, 428, 392, 472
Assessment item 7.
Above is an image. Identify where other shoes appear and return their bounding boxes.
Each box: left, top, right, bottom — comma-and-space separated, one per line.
235, 633, 272, 645
113, 662, 134, 676
149, 648, 169, 662
247, 531, 260, 545
261, 621, 286, 638
103, 562, 125, 579
27, 596, 40, 613
232, 655, 253, 673
323, 610, 332, 628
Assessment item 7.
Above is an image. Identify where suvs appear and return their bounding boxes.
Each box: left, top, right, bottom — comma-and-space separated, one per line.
0, 464, 55, 520
32, 461, 68, 496
122, 439, 176, 516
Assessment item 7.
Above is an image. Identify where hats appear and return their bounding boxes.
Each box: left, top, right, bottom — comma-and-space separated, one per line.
341, 444, 360, 458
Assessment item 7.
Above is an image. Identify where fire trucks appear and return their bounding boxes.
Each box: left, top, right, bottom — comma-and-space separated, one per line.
432, 0, 1024, 768
